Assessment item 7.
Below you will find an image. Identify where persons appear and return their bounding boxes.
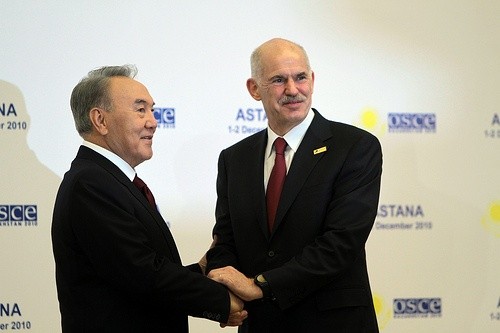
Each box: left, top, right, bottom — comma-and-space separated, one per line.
205, 37, 383, 333
50, 65, 245, 333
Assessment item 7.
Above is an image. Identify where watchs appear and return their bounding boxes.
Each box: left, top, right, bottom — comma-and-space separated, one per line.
253, 274, 270, 303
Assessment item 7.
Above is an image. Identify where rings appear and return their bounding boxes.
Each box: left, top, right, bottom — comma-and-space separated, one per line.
218, 271, 223, 279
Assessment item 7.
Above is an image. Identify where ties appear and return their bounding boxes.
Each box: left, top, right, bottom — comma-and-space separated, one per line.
133, 173, 157, 208
265, 138, 287, 234
